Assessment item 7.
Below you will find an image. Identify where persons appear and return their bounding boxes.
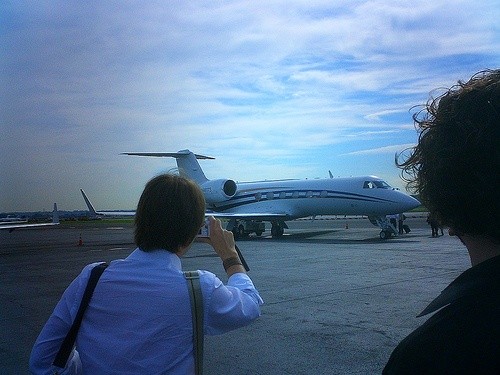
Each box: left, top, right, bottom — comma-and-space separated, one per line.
28, 167, 264, 375
390, 212, 407, 235
379, 68, 500, 375
427, 212, 444, 237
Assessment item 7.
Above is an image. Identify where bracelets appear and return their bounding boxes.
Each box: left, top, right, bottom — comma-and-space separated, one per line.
223, 256, 243, 273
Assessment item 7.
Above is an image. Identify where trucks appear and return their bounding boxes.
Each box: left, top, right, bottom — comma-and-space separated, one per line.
226, 219, 265, 237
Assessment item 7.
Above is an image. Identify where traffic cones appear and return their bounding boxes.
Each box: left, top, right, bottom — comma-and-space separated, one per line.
79, 233, 83, 246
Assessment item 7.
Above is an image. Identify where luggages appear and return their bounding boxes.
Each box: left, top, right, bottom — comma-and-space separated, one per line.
403, 224, 411, 233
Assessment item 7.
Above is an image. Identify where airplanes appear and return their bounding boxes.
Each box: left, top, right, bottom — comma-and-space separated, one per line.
0, 202, 60, 232
80, 149, 422, 240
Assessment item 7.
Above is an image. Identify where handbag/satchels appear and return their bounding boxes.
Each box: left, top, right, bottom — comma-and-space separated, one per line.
403, 215, 407, 220
